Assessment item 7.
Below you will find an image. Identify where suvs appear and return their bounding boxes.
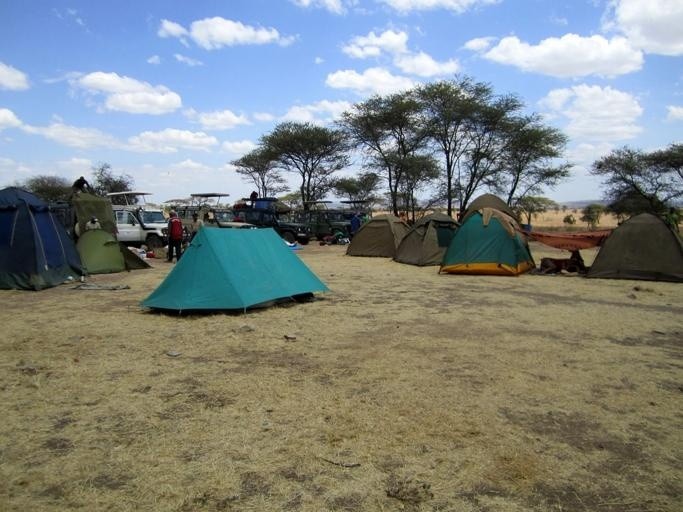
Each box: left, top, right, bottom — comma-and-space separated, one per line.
75, 191, 368, 251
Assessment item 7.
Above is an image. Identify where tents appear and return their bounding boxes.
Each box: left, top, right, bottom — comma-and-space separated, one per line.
456, 192, 520, 223
435, 206, 535, 277
344, 214, 407, 256
584, 211, 682, 283
133, 222, 335, 313
386, 211, 457, 268
0, 187, 85, 291
71, 226, 153, 273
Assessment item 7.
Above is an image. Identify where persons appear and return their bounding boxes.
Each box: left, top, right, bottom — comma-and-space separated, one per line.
190, 214, 203, 240
320, 213, 364, 245
84, 216, 101, 230
207, 208, 214, 223
399, 211, 408, 221
534, 256, 586, 275
163, 209, 183, 264
70, 176, 91, 193
232, 212, 243, 222
